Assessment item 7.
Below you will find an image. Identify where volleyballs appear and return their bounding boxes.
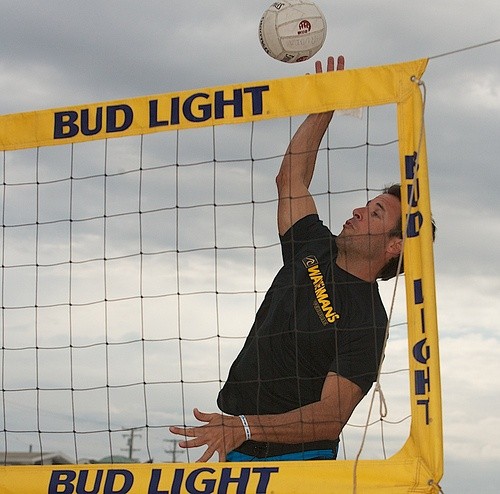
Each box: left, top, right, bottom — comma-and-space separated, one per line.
259, 1, 326, 63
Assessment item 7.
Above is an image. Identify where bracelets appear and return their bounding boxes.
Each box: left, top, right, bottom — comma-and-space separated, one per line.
239, 414, 252, 441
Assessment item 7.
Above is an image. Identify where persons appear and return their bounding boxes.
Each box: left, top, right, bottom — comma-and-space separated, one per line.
169, 55, 437, 465
